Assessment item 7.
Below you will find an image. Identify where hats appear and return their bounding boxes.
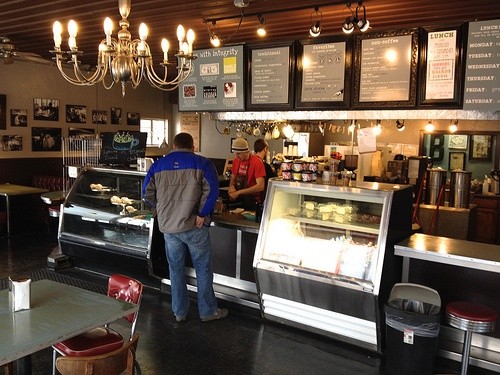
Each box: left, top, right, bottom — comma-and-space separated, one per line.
231, 138, 250, 153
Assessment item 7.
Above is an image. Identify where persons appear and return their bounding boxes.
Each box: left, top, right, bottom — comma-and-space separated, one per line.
92, 110, 108, 124
139, 132, 229, 325
253, 138, 279, 183
224, 81, 236, 94
228, 136, 266, 223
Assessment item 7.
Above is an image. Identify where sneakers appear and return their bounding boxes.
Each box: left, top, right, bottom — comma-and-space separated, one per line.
174, 300, 188, 321
201, 308, 229, 322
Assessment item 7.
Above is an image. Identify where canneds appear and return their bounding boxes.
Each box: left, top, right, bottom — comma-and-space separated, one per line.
281, 160, 319, 182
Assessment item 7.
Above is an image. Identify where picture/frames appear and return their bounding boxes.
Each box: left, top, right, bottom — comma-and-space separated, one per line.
448, 152, 466, 171
469, 135, 493, 162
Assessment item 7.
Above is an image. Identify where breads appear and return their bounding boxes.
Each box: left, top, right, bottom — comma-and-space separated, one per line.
91, 183, 135, 212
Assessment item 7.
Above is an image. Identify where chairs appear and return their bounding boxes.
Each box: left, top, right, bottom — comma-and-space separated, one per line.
52, 273, 143, 375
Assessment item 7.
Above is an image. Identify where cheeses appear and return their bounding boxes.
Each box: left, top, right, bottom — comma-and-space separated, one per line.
291, 201, 358, 223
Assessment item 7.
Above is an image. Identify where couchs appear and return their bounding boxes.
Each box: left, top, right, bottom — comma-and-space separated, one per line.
29, 176, 76, 224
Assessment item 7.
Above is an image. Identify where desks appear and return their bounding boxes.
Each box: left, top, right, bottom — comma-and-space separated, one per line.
394, 233, 500, 373
0, 279, 139, 375
0, 183, 50, 233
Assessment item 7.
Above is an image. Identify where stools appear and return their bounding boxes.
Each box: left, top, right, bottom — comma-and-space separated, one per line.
445, 301, 496, 375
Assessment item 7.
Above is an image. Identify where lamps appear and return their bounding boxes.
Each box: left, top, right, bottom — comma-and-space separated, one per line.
256, 14, 267, 36
309, 6, 322, 38
341, 0, 369, 34
159, 76, 169, 151
349, 120, 459, 135
94, 84, 102, 147
48, 0, 197, 97
208, 20, 220, 47
